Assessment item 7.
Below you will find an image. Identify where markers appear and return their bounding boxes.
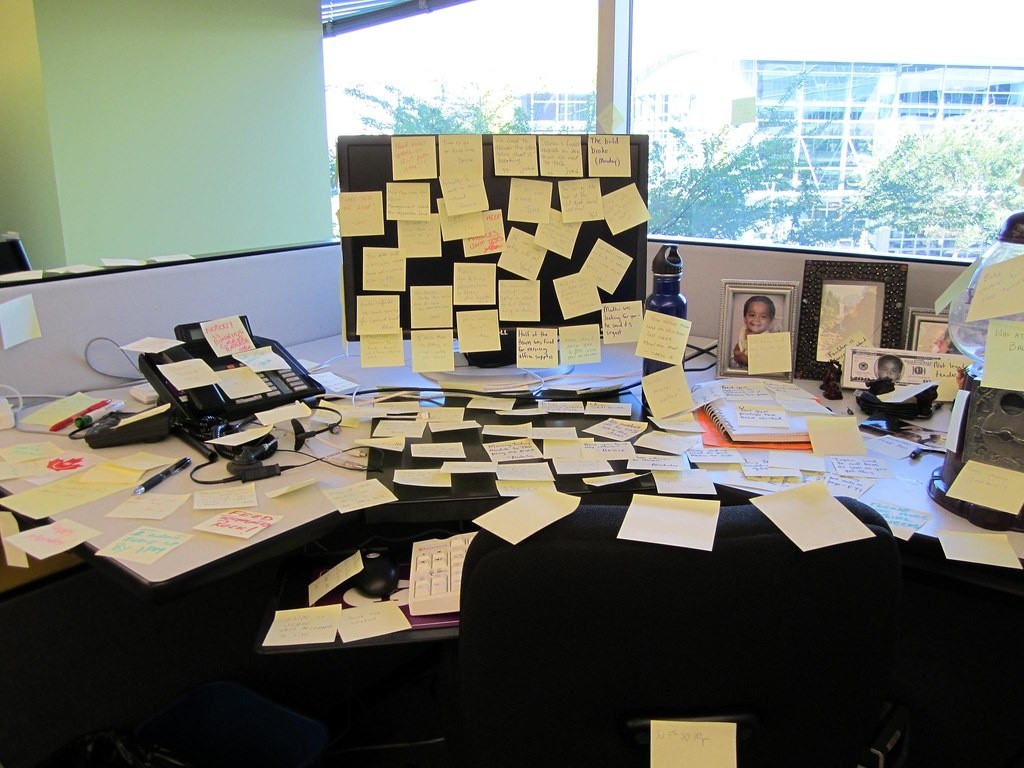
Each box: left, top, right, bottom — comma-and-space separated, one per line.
75, 398, 127, 429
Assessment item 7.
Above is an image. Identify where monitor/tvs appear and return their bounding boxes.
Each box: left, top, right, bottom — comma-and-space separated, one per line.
337, 134, 649, 387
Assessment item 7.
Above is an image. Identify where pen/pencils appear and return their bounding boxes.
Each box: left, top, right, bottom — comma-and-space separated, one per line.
49, 399, 113, 432
130, 457, 192, 497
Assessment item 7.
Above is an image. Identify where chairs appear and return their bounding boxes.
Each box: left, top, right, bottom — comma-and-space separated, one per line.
458, 495, 910, 768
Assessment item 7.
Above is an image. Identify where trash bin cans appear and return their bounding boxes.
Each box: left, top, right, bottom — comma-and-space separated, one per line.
30, 725, 190, 768
128, 680, 331, 768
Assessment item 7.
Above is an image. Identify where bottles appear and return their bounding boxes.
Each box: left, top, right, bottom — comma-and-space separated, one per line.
641, 244, 687, 408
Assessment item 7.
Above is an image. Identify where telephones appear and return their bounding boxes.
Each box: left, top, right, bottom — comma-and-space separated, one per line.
137, 313, 326, 429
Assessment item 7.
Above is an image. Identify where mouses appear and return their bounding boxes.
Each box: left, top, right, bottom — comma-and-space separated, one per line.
354, 552, 398, 597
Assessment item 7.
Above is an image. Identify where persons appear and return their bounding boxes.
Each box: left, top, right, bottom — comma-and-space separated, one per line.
733, 296, 775, 367
878, 356, 903, 383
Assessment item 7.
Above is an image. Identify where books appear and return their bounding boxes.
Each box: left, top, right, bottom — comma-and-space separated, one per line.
16, 392, 127, 436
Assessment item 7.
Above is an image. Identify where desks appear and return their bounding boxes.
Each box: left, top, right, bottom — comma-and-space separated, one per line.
0, 335, 1024, 768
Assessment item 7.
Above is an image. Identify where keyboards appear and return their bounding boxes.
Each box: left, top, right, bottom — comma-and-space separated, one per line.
408, 531, 479, 614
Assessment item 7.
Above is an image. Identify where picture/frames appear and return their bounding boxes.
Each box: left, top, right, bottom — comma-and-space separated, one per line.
905, 307, 965, 356
794, 260, 908, 383
714, 279, 800, 384
